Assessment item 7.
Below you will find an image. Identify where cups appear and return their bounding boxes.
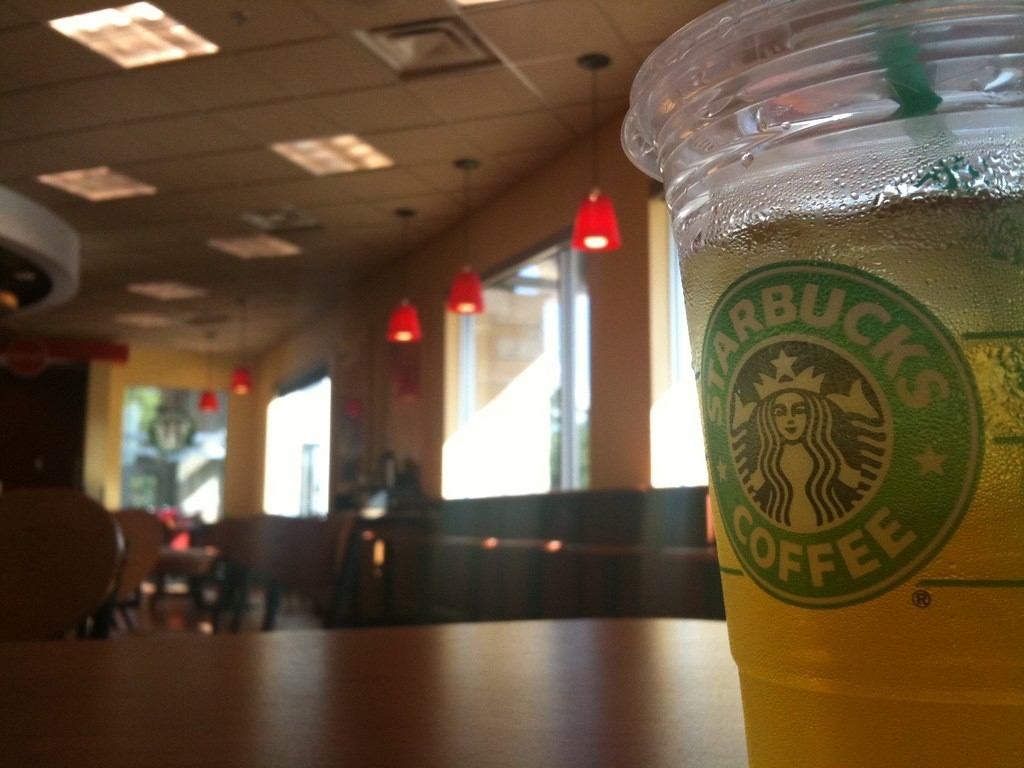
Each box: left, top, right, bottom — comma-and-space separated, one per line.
620, 0, 1024, 768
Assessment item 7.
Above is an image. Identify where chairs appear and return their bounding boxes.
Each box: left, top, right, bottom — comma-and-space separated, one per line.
0, 480, 172, 641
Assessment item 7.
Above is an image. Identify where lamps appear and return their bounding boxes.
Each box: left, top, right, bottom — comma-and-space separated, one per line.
386, 208, 422, 343
198, 330, 219, 415
446, 160, 485, 316
571, 52, 623, 252
229, 298, 254, 395
